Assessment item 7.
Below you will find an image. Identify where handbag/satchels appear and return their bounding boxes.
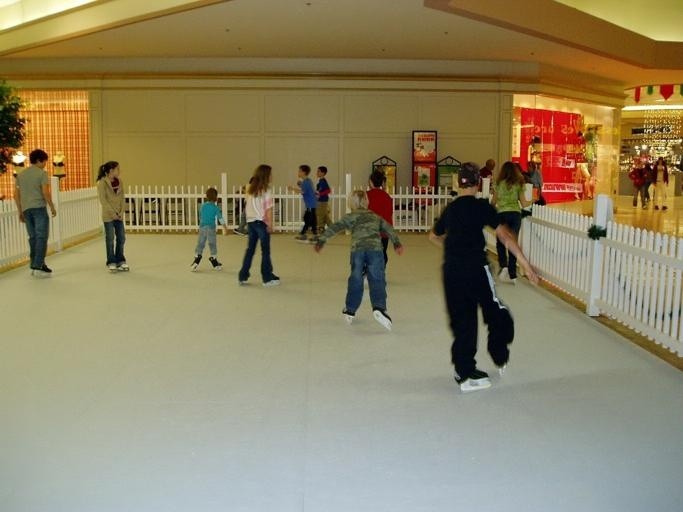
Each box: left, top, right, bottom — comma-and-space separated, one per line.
534, 184, 545, 206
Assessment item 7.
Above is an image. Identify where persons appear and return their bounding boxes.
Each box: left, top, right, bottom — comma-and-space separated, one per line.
314, 190, 402, 331
235, 163, 284, 287
527, 136, 542, 170
50, 150, 65, 182
231, 177, 254, 237
477, 158, 496, 193
93, 160, 127, 271
527, 162, 542, 198
285, 164, 316, 242
427, 162, 542, 390
10, 151, 27, 181
627, 153, 670, 212
192, 187, 227, 268
490, 160, 540, 285
365, 171, 392, 265
314, 166, 332, 233
571, 137, 593, 200
11, 149, 60, 278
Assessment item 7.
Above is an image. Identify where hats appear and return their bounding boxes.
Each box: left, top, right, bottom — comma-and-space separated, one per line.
457, 162, 480, 185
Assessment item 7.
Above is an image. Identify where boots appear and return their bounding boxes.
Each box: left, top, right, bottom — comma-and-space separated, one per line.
235, 229, 242, 233
373, 307, 392, 323
239, 272, 250, 282
30, 262, 52, 273
263, 273, 279, 283
107, 261, 129, 270
498, 266, 508, 275
191, 254, 202, 266
454, 368, 489, 384
655, 205, 659, 210
509, 273, 517, 279
496, 349, 509, 367
209, 257, 222, 268
662, 206, 667, 210
343, 308, 356, 318
295, 235, 321, 242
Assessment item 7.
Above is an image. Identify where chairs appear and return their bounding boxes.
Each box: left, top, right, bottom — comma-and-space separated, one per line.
122, 197, 285, 227
